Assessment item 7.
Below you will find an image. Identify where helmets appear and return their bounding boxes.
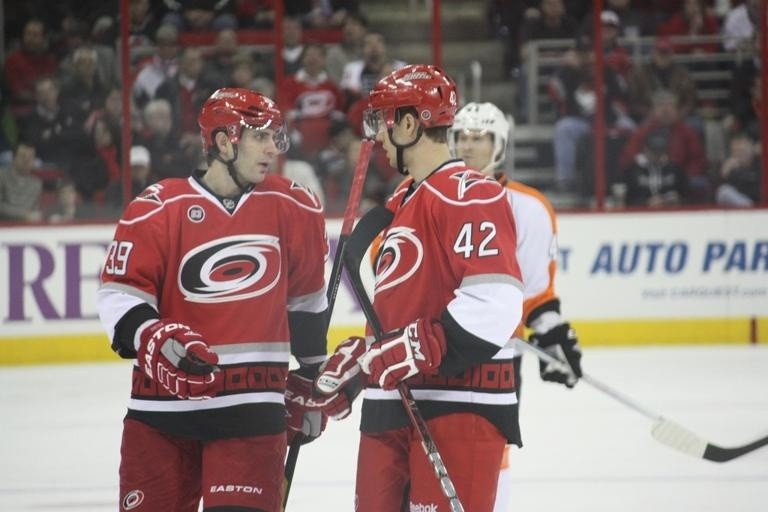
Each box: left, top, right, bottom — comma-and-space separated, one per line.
448, 101, 510, 167
197, 87, 284, 150
367, 65, 460, 131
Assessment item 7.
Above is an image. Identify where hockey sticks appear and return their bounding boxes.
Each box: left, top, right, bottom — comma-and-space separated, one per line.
506, 337, 768, 465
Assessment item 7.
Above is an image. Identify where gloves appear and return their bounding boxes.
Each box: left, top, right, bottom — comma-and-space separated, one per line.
137, 317, 219, 402
528, 311, 583, 388
315, 334, 369, 422
283, 370, 329, 446
358, 317, 447, 391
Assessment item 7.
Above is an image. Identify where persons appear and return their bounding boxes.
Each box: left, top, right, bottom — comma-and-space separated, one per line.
309, 58, 529, 512
90, 83, 337, 512
444, 98, 586, 473
2, 0, 768, 229
